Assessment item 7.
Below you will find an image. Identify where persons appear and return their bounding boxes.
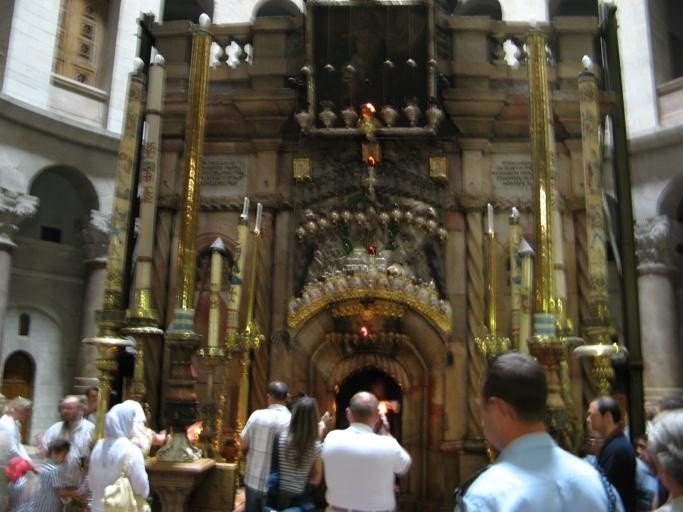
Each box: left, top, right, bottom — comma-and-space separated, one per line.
263, 395, 324, 511
236, 380, 329, 511
453, 353, 625, 512
322, 391, 411, 512
570, 392, 683, 512
0, 380, 169, 511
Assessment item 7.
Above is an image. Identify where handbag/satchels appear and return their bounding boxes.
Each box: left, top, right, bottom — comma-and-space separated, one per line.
100, 476, 152, 512
260, 492, 322, 512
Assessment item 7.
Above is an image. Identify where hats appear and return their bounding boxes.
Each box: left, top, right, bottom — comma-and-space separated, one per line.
4, 457, 30, 481
